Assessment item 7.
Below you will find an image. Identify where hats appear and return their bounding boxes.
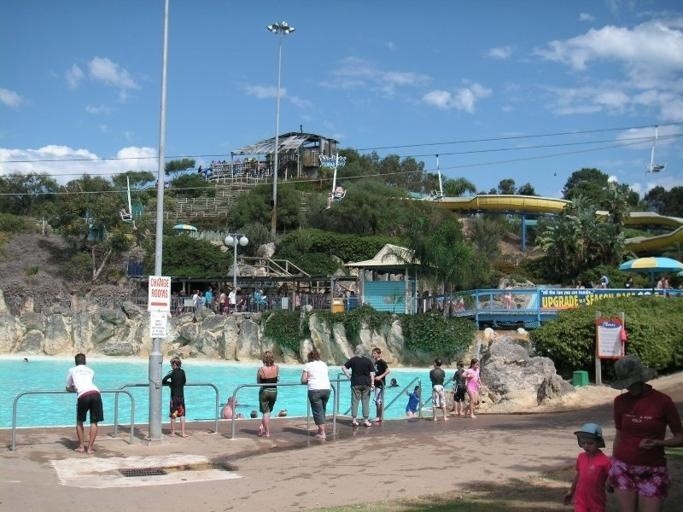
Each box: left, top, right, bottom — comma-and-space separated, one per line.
608, 355, 659, 390
574, 423, 606, 447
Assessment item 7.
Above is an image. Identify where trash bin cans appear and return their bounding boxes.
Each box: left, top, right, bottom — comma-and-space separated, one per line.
572, 371, 588, 386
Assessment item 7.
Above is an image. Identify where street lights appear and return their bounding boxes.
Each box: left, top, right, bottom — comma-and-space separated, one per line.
224, 232, 249, 314
266, 20, 296, 237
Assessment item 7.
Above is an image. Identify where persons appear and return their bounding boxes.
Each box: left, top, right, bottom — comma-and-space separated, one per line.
121, 208, 137, 230
172, 286, 267, 314
162, 357, 188, 437
606, 355, 683, 512
576, 275, 671, 289
504, 283, 512, 309
563, 423, 613, 512
326, 187, 344, 209
66, 354, 104, 454
221, 344, 482, 439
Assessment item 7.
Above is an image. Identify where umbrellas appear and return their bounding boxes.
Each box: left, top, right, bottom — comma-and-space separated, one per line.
172, 224, 198, 237
619, 256, 683, 288
